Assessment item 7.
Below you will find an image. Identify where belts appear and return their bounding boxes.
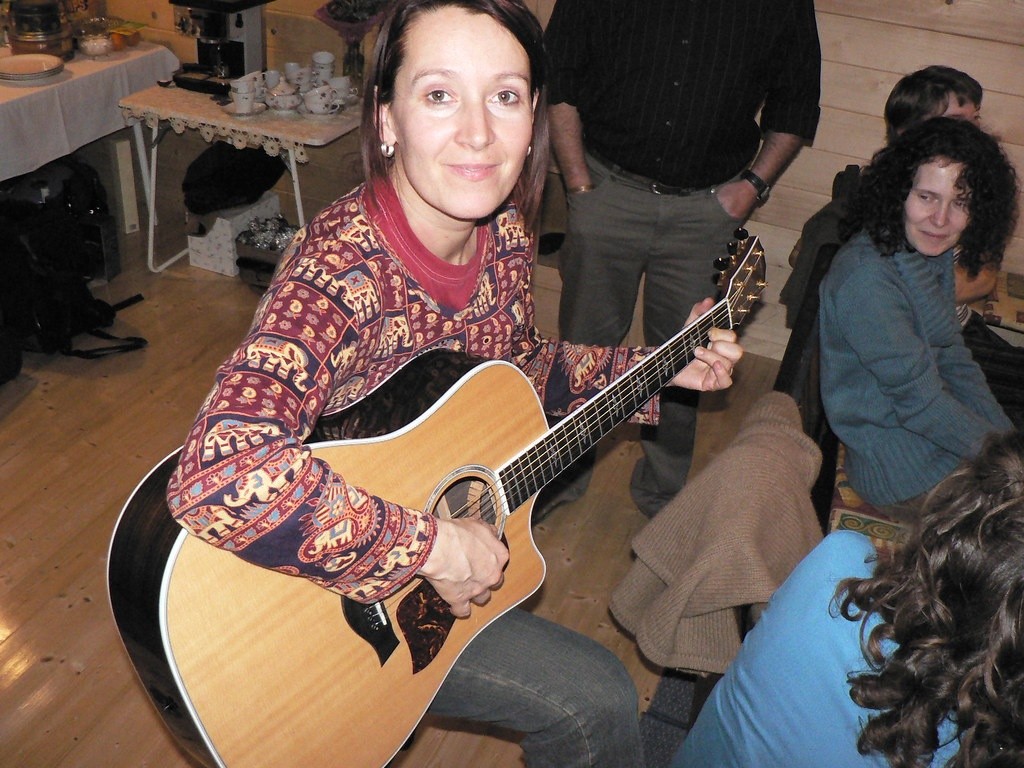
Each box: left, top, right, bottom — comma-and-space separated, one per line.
582, 144, 690, 197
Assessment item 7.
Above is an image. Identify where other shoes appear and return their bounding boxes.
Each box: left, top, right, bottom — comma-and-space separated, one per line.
531, 482, 594, 521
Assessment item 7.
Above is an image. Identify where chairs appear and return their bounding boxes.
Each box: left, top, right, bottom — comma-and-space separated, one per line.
685, 164, 1023, 729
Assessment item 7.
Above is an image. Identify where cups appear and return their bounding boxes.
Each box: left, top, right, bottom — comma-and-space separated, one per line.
228, 52, 357, 114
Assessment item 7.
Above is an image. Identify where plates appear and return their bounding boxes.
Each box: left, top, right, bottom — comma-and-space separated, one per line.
222, 102, 266, 121
0, 53, 64, 85
298, 104, 337, 120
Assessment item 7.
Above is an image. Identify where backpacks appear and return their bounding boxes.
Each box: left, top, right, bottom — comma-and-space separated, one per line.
2, 199, 149, 357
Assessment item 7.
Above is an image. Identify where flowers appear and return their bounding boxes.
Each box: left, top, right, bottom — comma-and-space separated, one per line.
312, 0, 400, 48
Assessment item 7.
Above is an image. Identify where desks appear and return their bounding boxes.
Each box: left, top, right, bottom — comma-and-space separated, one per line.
0, 38, 179, 233
120, 83, 362, 273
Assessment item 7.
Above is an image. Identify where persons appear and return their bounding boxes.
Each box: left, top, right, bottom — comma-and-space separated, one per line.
819, 118, 1024, 521
668, 431, 1024, 768
529, 0, 822, 534
883, 66, 1024, 439
165, 0, 744, 768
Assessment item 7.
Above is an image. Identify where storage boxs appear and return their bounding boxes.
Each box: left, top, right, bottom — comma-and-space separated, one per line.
185, 190, 300, 288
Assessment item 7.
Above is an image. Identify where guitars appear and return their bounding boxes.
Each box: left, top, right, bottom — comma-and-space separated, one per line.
100, 226, 770, 768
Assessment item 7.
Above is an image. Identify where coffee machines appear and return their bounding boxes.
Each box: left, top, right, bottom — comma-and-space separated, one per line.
169, 0, 274, 95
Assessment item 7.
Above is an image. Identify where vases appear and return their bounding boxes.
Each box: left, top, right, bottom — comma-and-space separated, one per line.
343, 41, 364, 97
